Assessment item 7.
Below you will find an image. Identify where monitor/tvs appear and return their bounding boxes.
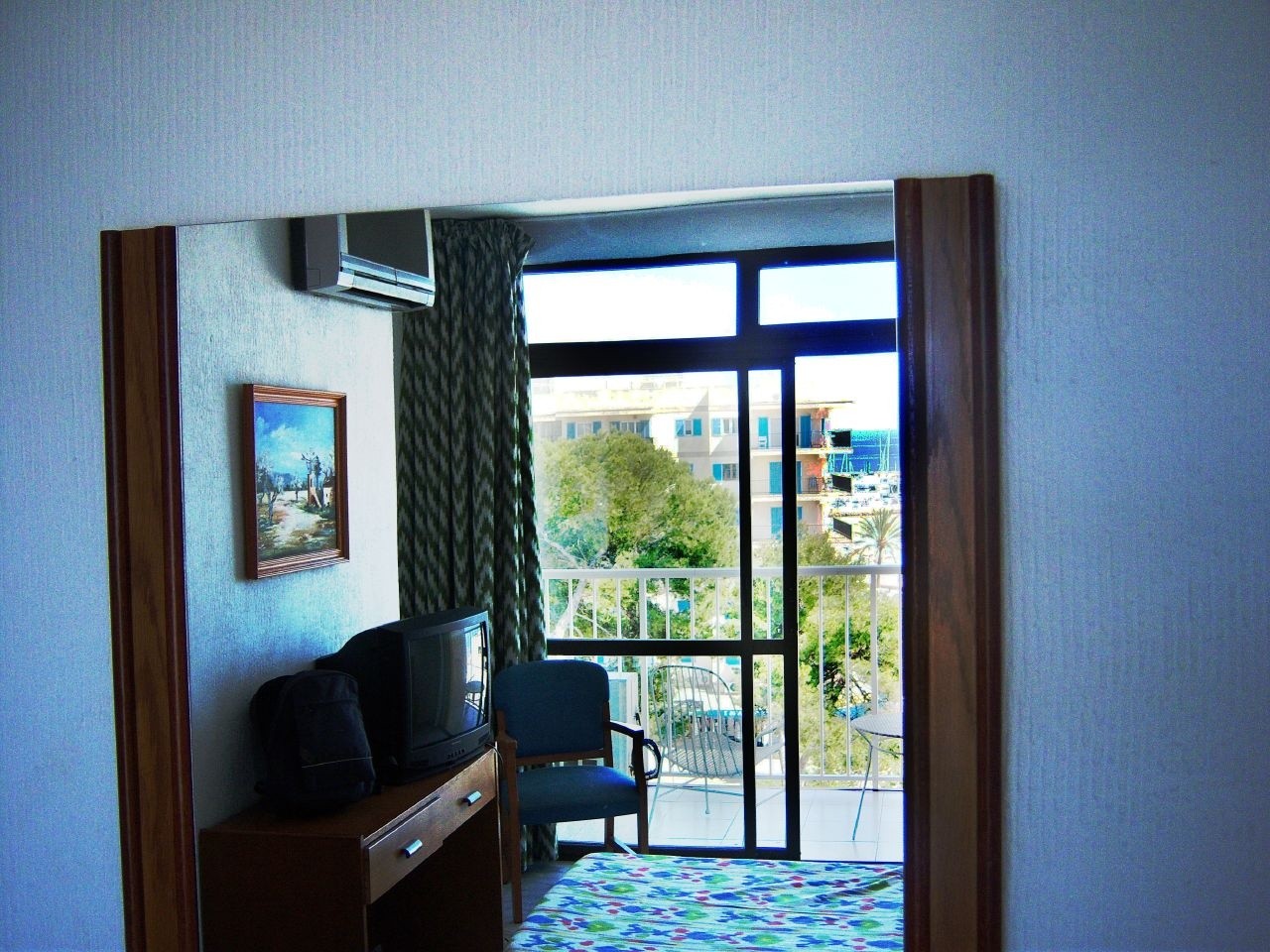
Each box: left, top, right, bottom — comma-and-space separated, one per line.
312, 609, 495, 786
306, 209, 437, 313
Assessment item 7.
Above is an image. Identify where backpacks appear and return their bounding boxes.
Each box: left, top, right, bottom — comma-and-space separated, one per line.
248, 668, 377, 817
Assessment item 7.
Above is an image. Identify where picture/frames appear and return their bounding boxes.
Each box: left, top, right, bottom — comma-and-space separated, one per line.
241, 383, 350, 579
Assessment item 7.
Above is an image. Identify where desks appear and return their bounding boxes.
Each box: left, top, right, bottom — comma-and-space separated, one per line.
850, 711, 904, 840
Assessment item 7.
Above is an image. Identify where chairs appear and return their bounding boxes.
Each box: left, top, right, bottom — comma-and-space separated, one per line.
495, 660, 649, 925
648, 665, 786, 824
808, 476, 819, 493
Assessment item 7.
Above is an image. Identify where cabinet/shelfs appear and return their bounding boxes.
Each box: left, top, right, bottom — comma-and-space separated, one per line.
200, 746, 506, 952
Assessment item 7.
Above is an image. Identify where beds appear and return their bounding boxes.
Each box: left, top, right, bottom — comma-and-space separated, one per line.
507, 853, 905, 952
